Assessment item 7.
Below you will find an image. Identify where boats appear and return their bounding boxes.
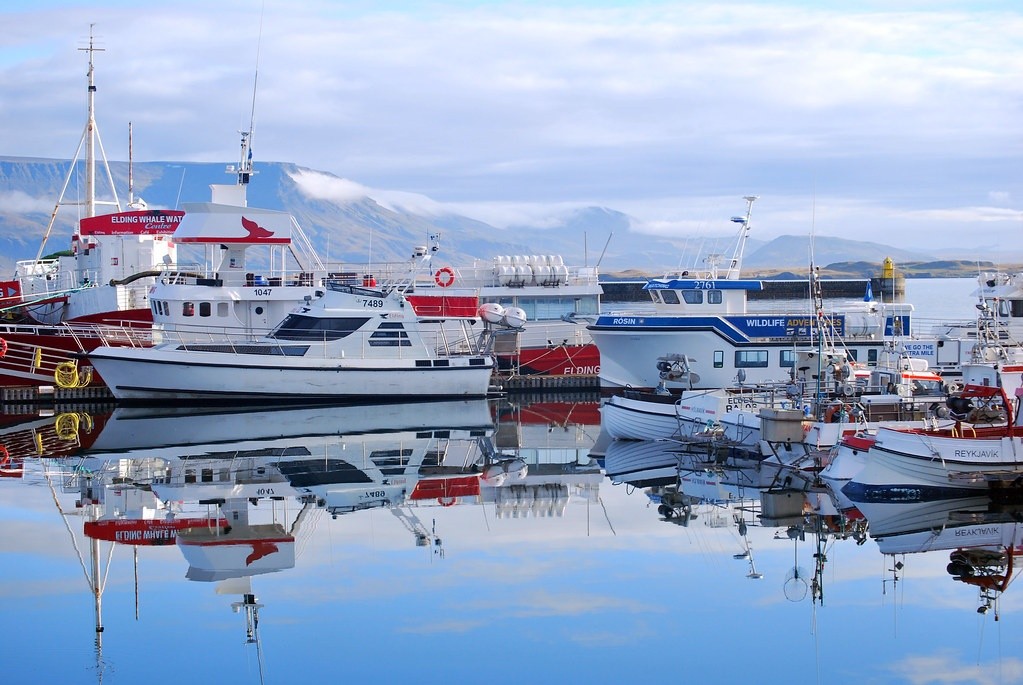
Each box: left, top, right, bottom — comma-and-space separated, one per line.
0, 19, 1023, 489
0, 396, 1023, 684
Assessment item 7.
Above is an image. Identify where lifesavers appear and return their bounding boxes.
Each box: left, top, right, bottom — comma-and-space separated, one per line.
435, 268, 454, 287
0, 337, 7, 357
824, 515, 856, 532
0, 446, 8, 464
825, 403, 856, 423
438, 497, 456, 507
